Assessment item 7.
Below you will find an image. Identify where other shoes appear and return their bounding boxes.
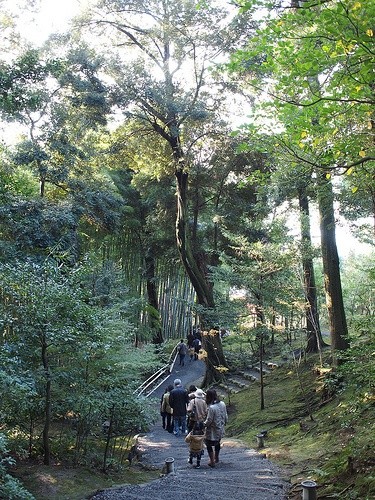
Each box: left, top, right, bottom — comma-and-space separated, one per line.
208, 461, 215, 467
214, 458, 219, 462
185, 430, 188, 434
188, 458, 193, 464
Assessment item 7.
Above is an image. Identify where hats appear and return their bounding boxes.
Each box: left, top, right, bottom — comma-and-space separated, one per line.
195, 389, 205, 397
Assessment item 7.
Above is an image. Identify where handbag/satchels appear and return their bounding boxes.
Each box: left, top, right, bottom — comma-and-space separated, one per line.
188, 399, 195, 418
201, 450, 204, 455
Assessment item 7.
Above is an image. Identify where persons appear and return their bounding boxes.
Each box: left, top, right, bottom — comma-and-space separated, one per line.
184, 423, 206, 468
160, 378, 207, 437
188, 326, 203, 360
177, 340, 187, 366
203, 389, 229, 468
